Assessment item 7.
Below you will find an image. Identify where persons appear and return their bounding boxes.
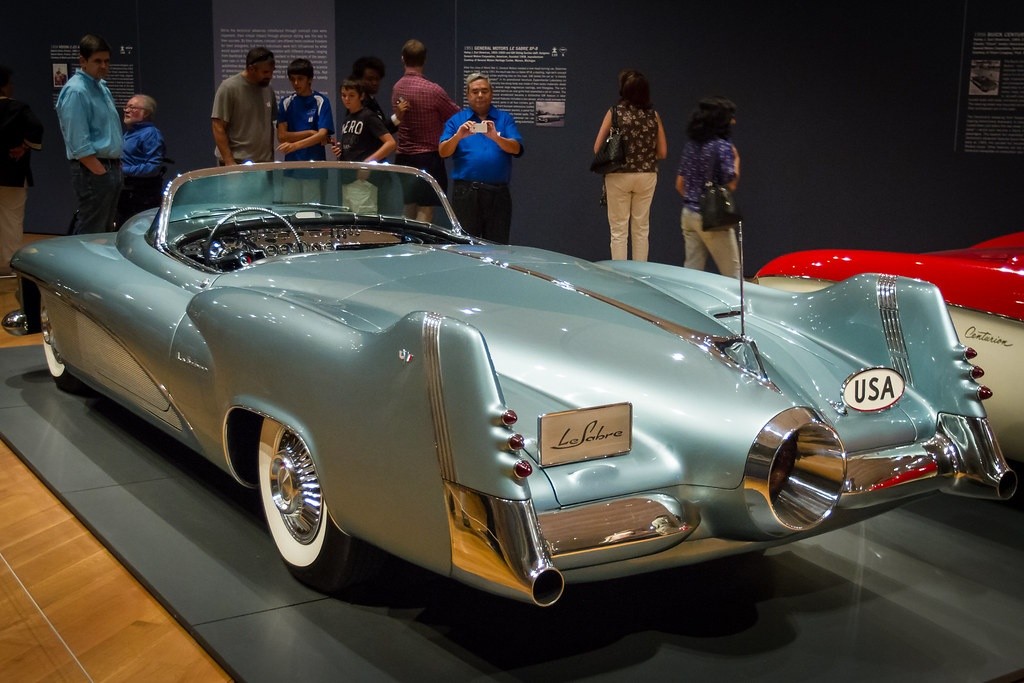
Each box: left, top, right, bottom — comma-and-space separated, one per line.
391, 38, 462, 226
275, 57, 336, 205
675, 96, 744, 279
0, 66, 46, 278
593, 70, 667, 264
209, 47, 279, 202
328, 55, 413, 215
54, 33, 125, 234
65, 93, 166, 238
437, 71, 525, 248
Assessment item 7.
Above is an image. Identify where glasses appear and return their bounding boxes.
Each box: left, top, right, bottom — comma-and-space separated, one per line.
123, 106, 145, 112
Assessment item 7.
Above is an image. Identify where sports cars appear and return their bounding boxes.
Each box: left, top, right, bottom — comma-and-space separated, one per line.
1, 161, 1024, 607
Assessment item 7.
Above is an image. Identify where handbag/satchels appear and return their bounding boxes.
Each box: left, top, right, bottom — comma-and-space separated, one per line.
590, 105, 626, 173
702, 139, 741, 231
342, 179, 378, 215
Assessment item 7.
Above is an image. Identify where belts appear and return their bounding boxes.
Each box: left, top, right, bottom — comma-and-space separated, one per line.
454, 179, 507, 192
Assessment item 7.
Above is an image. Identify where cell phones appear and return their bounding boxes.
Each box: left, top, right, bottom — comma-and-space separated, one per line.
470, 123, 488, 133
332, 137, 337, 146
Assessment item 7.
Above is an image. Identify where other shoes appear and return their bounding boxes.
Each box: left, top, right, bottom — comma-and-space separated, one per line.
0, 272, 17, 278
